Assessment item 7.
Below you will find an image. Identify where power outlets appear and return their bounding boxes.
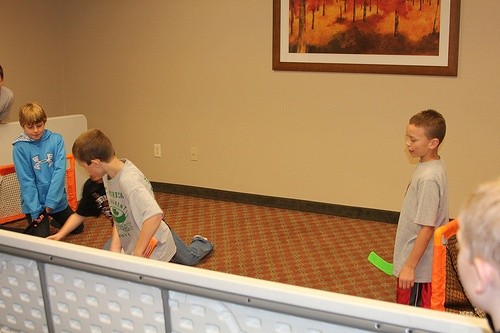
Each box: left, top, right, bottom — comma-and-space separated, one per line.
191, 148, 197, 160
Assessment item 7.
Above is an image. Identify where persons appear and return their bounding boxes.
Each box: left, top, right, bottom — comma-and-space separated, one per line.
0, 65, 15, 125
72, 127, 178, 262
12, 103, 84, 237
48, 174, 214, 265
456, 182, 500, 332
392, 110, 449, 309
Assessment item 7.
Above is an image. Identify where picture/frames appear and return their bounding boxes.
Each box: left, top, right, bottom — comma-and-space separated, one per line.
272, 0, 461, 76
